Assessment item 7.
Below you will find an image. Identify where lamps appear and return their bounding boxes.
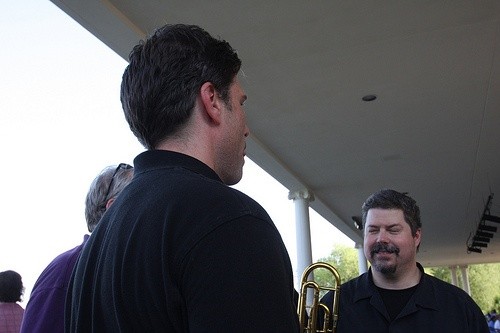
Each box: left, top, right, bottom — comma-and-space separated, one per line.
466, 192, 500, 254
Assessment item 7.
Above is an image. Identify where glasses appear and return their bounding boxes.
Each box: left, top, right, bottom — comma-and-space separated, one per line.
103, 163, 133, 203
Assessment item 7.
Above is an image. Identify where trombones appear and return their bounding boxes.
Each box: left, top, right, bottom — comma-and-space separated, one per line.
298, 262, 341, 333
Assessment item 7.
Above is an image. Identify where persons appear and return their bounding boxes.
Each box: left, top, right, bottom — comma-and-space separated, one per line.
19, 163, 134, 332
309, 191, 491, 332
0, 270, 25, 333
64, 22, 304, 332
